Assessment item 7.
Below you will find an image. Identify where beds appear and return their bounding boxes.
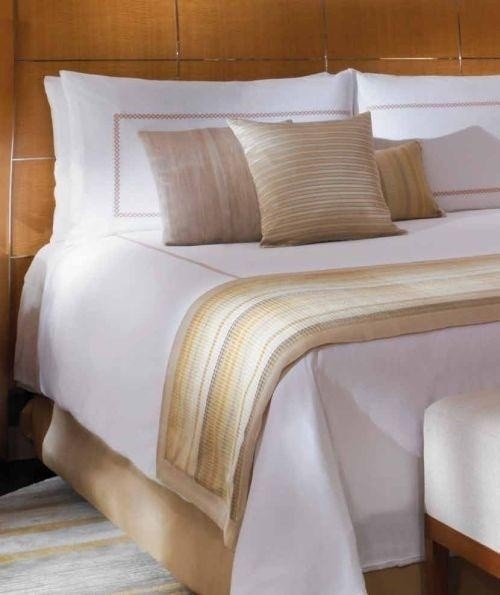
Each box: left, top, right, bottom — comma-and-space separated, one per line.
0, 65, 500, 594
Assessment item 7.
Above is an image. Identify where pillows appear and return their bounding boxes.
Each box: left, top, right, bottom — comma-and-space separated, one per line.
39, 67, 497, 250
